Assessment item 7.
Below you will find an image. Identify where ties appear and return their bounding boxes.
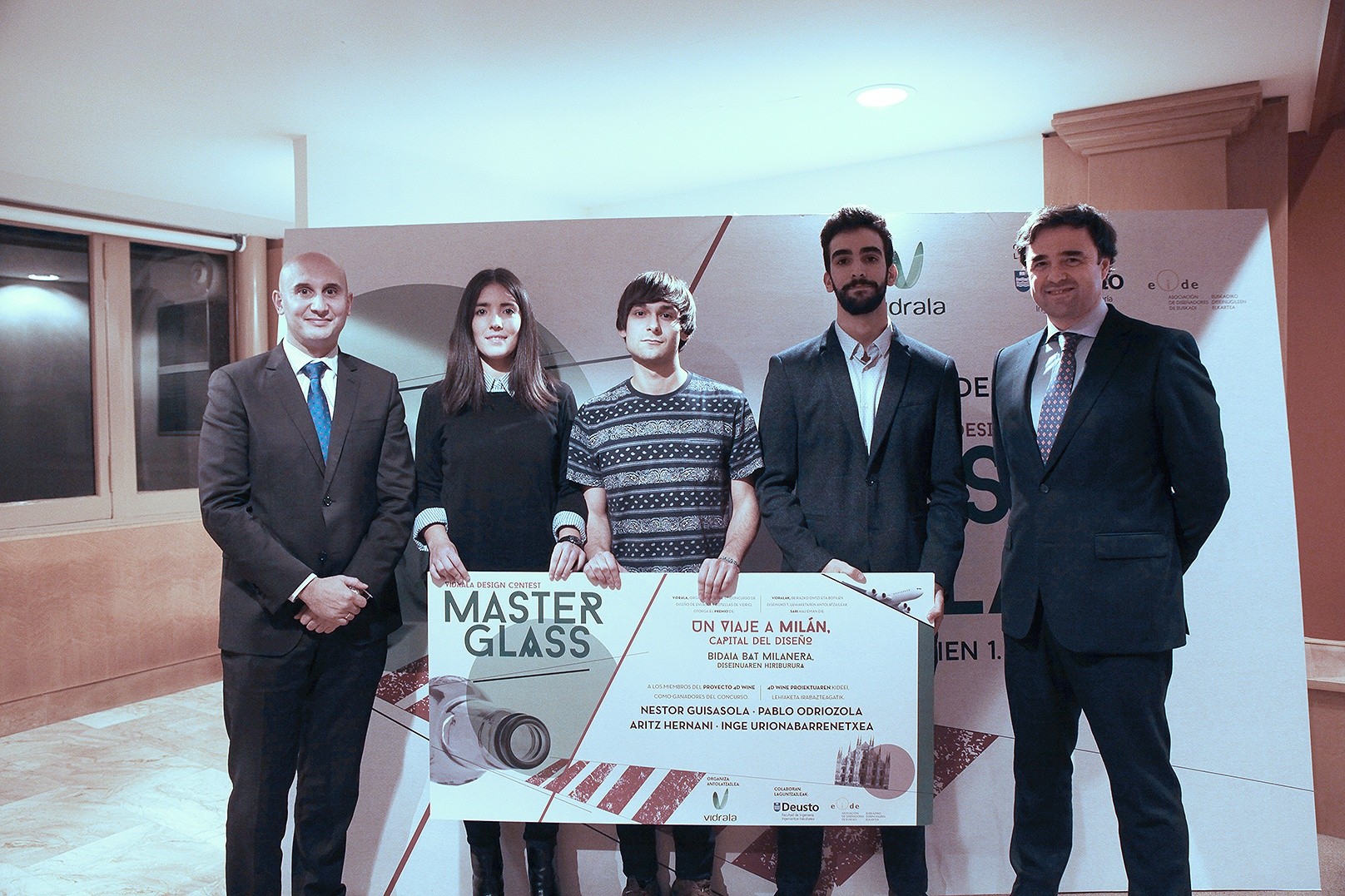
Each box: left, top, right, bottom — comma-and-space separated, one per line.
300, 361, 332, 467
1037, 332, 1085, 466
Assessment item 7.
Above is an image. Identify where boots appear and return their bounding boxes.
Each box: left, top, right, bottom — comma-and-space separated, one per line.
470, 851, 504, 896
526, 839, 561, 896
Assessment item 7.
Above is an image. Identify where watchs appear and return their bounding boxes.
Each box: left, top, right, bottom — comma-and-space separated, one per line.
558, 535, 583, 549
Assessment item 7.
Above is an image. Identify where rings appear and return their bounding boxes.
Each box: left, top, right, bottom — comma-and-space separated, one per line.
345, 613, 351, 620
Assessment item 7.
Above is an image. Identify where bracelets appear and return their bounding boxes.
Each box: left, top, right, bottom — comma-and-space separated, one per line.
719, 557, 739, 567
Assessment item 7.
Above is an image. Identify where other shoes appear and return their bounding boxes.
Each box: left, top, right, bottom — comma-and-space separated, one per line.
671, 879, 713, 896
622, 876, 660, 896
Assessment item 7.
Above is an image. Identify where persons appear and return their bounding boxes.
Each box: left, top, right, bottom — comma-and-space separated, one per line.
198, 253, 415, 896
411, 268, 587, 896
991, 203, 1231, 896
566, 271, 764, 896
758, 210, 969, 896
